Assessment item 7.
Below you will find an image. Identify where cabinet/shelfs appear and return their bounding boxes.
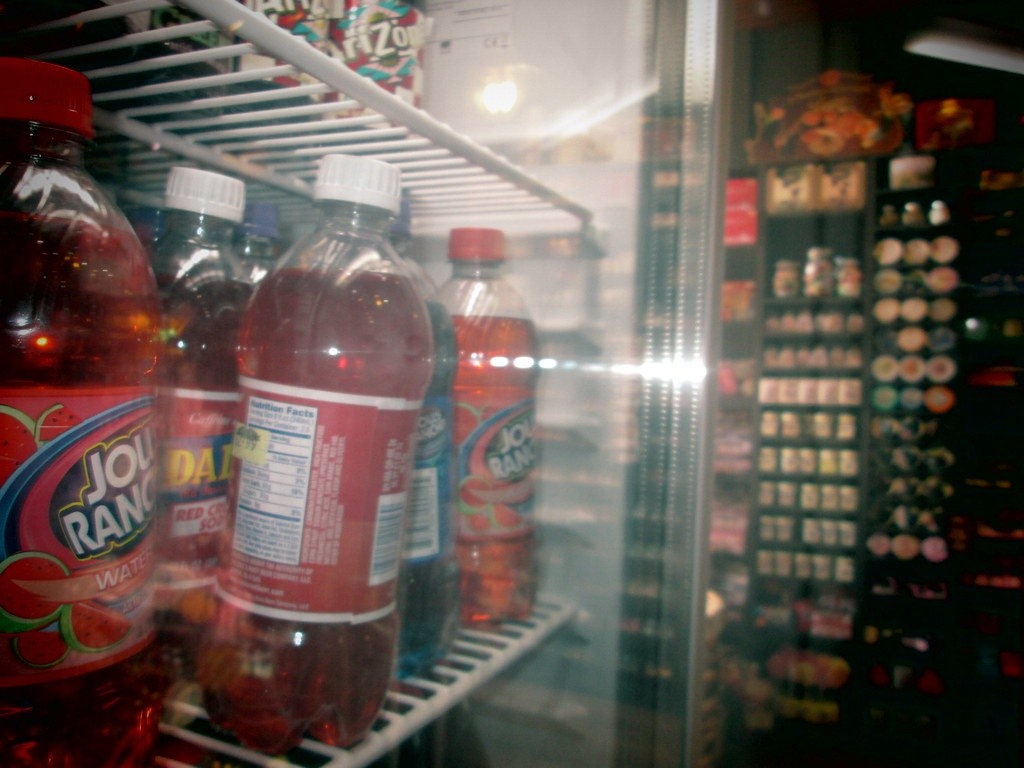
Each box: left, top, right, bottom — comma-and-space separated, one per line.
616, 150, 1024, 767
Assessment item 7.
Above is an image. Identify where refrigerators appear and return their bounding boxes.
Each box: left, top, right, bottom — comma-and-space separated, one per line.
0, 0, 707, 768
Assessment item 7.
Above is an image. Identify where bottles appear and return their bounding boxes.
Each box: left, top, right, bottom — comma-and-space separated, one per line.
0, 45, 562, 768
71, 0, 431, 163
